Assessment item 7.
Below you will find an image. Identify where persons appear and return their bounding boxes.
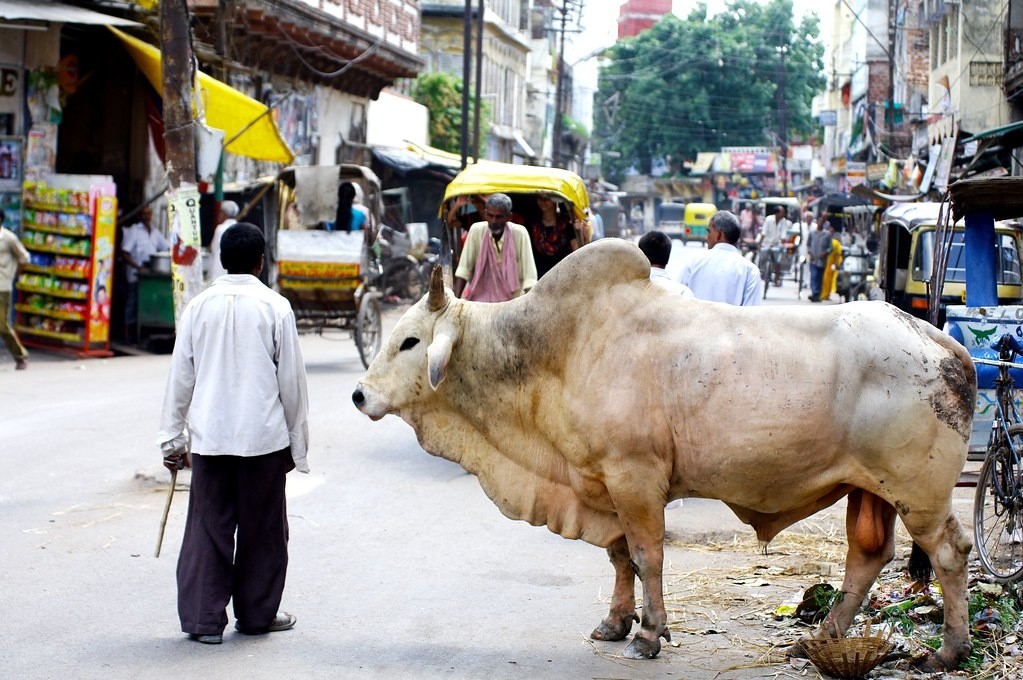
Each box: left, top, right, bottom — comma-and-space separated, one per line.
676, 210, 761, 306
122, 206, 170, 346
156, 223, 309, 644
0, 208, 31, 369
455, 192, 538, 303
739, 202, 842, 302
206, 201, 239, 281
285, 182, 376, 230
526, 193, 579, 281
447, 193, 525, 232
583, 204, 643, 243
638, 230, 695, 298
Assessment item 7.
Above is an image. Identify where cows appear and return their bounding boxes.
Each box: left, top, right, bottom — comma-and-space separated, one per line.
351, 239, 978, 667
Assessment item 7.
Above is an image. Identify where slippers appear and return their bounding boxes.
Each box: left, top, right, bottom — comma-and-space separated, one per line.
235, 613, 297, 634
190, 632, 223, 644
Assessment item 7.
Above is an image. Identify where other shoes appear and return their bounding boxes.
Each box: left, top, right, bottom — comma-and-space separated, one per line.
809, 294, 820, 303
14, 355, 28, 369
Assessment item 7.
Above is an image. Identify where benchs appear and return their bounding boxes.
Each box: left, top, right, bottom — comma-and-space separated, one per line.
943, 305, 1023, 446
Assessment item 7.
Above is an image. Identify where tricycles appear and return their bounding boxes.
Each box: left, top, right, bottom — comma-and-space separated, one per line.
749, 197, 806, 300
274, 164, 386, 370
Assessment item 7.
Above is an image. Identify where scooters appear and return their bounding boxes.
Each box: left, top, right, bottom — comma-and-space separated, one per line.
832, 246, 872, 302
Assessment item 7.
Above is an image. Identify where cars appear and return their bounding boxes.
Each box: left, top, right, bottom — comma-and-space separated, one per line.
657, 203, 685, 240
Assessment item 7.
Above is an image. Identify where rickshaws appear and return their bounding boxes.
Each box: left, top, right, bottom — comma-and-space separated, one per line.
438, 161, 596, 303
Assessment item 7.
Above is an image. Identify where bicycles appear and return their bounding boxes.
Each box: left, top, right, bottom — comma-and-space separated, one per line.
848, 279, 877, 303
967, 334, 1023, 584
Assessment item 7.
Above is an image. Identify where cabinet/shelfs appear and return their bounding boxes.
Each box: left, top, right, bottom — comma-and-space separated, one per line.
0, 135, 117, 359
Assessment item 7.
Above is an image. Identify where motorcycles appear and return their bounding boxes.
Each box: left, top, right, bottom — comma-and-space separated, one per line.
879, 204, 1023, 331
682, 203, 717, 247
